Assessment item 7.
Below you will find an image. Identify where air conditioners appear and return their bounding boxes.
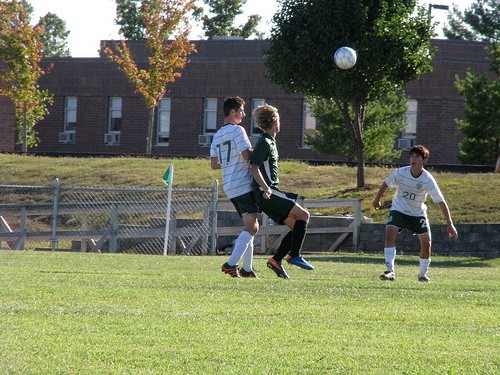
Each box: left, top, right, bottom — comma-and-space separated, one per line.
58, 132, 75, 142
104, 133, 119, 144
198, 134, 213, 145
397, 138, 415, 149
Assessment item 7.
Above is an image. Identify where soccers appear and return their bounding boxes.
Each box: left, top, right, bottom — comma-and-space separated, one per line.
334, 46, 357, 69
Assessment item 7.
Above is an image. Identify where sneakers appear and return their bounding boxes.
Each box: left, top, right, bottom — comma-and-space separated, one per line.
221, 263, 243, 278
418, 274, 429, 281
286, 255, 314, 270
380, 270, 395, 280
239, 267, 258, 278
267, 258, 290, 279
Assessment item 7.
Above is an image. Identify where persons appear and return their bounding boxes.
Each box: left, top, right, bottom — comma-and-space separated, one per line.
209, 95, 259, 279
250, 103, 314, 279
372, 145, 458, 282
